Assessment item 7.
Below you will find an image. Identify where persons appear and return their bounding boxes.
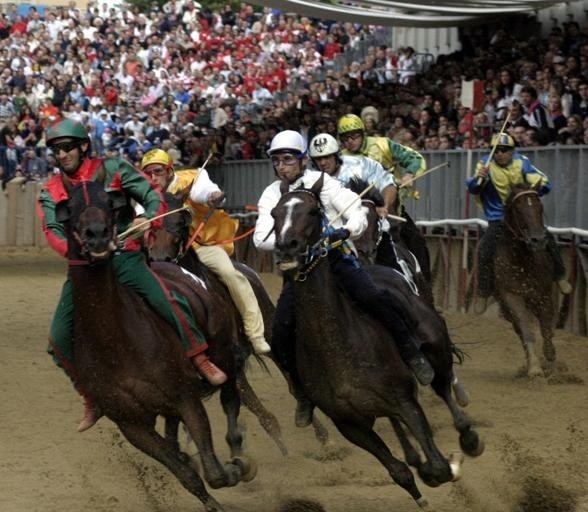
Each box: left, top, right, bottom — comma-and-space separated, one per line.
134, 148, 272, 354
308, 111, 434, 303
467, 133, 572, 313
1, 1, 588, 194
251, 130, 434, 427
35, 121, 227, 431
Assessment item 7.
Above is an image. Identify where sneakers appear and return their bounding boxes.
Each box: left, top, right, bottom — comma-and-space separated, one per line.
248, 337, 271, 355
473, 295, 487, 315
556, 279, 572, 294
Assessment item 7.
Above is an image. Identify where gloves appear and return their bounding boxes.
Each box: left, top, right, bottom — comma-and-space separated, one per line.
331, 227, 350, 242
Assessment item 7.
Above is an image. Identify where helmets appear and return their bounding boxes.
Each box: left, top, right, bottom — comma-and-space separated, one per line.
337, 114, 366, 135
141, 148, 174, 169
46, 119, 89, 146
308, 133, 339, 158
266, 129, 307, 154
490, 132, 515, 147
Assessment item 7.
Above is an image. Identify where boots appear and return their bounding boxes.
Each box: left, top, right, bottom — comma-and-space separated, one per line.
397, 337, 435, 385
77, 394, 105, 432
192, 353, 228, 386
289, 384, 317, 427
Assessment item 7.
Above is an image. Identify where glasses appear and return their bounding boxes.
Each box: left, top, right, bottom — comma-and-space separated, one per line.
491, 146, 513, 153
144, 160, 173, 176
339, 130, 367, 143
271, 149, 307, 167
50, 140, 87, 153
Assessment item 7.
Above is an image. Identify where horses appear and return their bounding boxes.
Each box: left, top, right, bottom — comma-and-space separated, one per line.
48, 179, 255, 512
268, 170, 462, 512
463, 176, 562, 392
343, 175, 482, 459
146, 178, 330, 460
